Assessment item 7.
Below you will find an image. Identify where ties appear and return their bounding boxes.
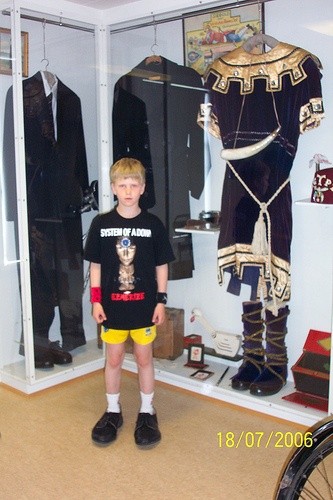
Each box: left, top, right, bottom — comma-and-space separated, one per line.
47, 93, 55, 143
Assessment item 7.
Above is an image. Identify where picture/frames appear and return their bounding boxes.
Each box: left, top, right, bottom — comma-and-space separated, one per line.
0, 27, 28, 77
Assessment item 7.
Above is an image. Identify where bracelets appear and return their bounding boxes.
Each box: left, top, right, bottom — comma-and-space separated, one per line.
155, 292, 168, 304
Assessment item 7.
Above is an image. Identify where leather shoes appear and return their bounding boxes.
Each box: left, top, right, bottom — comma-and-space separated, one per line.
135, 413, 161, 444
35, 350, 52, 368
47, 341, 73, 363
92, 411, 122, 443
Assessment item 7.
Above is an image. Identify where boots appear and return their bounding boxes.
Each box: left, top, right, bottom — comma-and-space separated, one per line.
250, 307, 289, 395
231, 301, 265, 391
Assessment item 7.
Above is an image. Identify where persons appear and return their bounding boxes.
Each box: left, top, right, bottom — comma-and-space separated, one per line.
82, 156, 177, 449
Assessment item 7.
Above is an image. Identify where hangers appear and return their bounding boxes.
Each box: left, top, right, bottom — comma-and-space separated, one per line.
212, 21, 312, 70
119, 44, 198, 87
9, 59, 81, 100
